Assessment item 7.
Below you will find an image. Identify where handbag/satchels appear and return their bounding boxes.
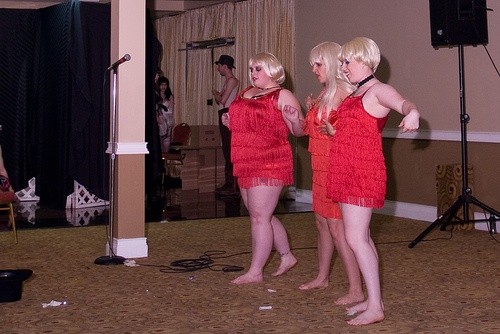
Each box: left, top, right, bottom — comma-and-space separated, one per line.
171, 123, 191, 146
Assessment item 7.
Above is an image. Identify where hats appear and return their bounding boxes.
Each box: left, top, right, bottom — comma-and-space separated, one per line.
215, 55, 238, 70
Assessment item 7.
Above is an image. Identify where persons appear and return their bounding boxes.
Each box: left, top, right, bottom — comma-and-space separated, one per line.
221, 50, 307, 284
153, 69, 175, 157
298, 40, 366, 305
319, 35, 420, 327
210, 54, 241, 196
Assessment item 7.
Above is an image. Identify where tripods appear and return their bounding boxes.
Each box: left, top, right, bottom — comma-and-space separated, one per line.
407, 46, 500, 248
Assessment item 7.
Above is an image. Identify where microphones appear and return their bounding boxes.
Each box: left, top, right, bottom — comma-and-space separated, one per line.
106, 54, 131, 71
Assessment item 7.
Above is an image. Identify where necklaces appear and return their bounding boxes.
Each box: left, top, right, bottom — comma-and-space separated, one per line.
257, 86, 280, 91
357, 74, 375, 88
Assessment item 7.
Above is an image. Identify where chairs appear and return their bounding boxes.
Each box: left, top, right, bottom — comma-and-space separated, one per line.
162, 120, 191, 170
0, 174, 18, 244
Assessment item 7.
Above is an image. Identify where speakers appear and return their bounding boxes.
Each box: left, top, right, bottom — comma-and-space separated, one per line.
429, 0, 488, 50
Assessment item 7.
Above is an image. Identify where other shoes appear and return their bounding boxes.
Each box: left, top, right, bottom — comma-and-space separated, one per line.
215, 187, 236, 195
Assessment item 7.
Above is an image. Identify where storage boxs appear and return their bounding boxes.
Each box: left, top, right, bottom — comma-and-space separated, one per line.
191, 124, 220, 147
436, 164, 474, 230
